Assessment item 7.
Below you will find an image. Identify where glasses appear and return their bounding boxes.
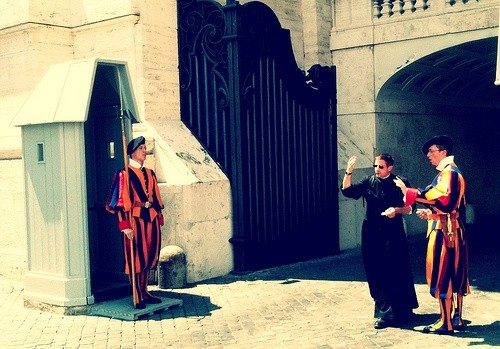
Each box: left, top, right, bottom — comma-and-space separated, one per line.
373, 164, 384, 169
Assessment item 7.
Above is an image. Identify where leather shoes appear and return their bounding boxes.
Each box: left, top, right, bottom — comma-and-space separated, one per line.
374, 318, 387, 328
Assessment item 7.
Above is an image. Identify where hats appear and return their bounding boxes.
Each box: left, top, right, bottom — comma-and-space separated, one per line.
422, 135, 453, 154
127, 136, 145, 155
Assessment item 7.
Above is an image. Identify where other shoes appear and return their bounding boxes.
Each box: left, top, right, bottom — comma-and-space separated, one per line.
143, 296, 162, 303
450, 317, 464, 330
132, 299, 145, 309
422, 318, 454, 335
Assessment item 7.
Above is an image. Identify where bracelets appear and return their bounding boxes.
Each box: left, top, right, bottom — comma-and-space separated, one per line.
345, 172, 352, 175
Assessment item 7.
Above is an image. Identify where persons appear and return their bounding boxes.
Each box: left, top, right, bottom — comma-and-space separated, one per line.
393, 134, 471, 335
105, 136, 164, 309
340, 153, 420, 329
